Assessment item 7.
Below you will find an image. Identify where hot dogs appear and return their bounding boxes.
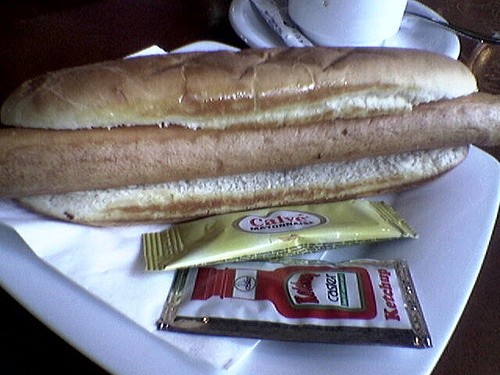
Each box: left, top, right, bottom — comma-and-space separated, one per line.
1, 48, 499, 229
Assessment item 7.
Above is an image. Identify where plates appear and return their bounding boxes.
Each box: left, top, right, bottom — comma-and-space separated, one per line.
229, 0, 461, 60
0, 41, 500, 374
